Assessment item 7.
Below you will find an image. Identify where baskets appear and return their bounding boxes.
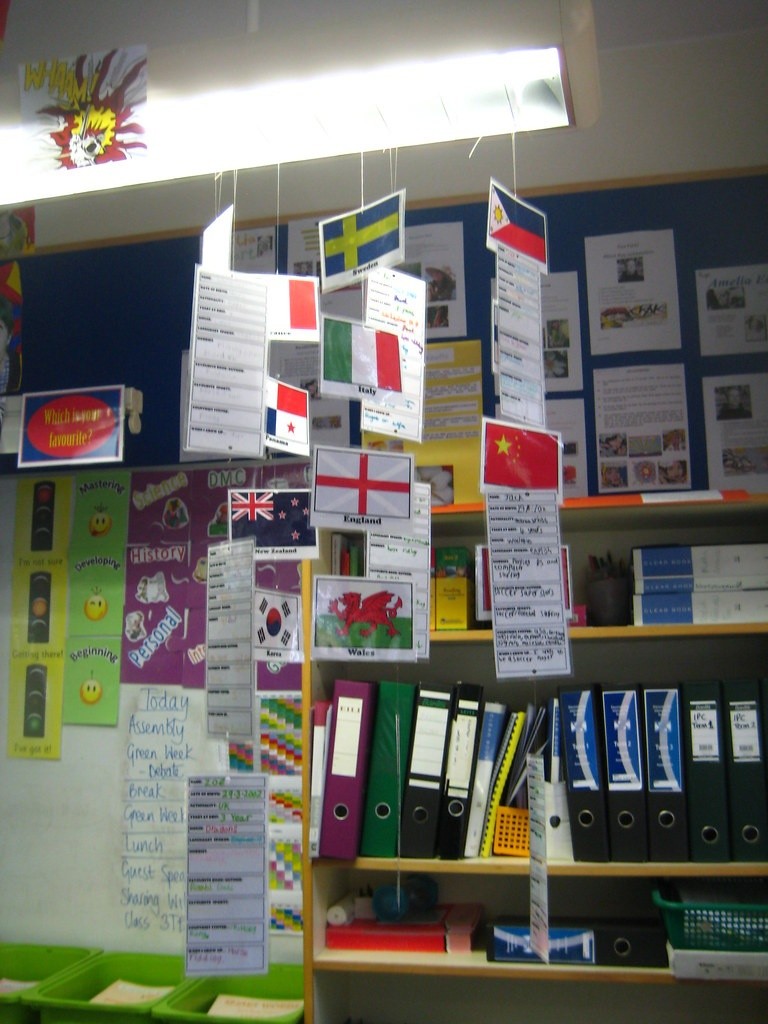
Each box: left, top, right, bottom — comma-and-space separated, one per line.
651, 878, 768, 952
493, 804, 530, 856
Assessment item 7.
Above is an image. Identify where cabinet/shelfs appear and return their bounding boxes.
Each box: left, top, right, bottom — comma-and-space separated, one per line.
301, 490, 768, 1023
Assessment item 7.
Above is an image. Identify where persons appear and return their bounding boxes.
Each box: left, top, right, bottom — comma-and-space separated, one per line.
601, 431, 686, 486
718, 388, 751, 418
618, 260, 645, 282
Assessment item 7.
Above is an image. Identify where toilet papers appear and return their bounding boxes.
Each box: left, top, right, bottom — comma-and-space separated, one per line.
327, 895, 354, 926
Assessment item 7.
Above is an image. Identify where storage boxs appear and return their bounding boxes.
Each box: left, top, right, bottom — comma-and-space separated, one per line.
0, 941, 306, 1024
308, 543, 768, 968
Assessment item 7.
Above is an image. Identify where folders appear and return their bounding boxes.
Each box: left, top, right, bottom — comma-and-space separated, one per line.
486, 917, 669, 967
464, 702, 506, 857
599, 687, 649, 862
558, 686, 610, 863
321, 678, 373, 858
359, 681, 418, 859
680, 679, 732, 862
641, 686, 690, 862
308, 700, 329, 858
399, 682, 456, 859
440, 684, 483, 858
722, 679, 768, 861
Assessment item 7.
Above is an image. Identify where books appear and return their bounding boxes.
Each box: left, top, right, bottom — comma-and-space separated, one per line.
325, 903, 767, 981
629, 543, 768, 627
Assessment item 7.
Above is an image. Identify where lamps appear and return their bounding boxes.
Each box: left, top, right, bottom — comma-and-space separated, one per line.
1, 0, 579, 207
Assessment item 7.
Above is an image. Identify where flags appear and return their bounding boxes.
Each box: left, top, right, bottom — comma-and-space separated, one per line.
252, 279, 317, 331
322, 317, 403, 392
313, 448, 411, 519
230, 491, 317, 547
265, 377, 308, 444
322, 194, 400, 277
484, 422, 559, 490
489, 183, 546, 264
